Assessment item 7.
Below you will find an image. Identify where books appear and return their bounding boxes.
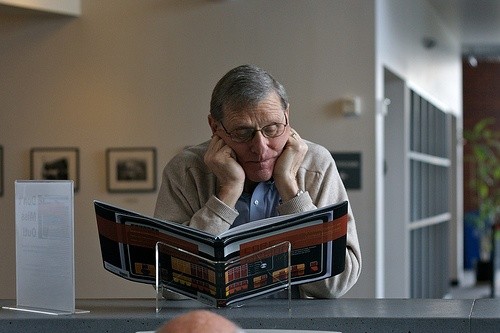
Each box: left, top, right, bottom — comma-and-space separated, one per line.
94, 200, 348, 307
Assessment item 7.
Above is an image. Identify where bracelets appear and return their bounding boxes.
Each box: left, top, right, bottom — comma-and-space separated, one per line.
279, 189, 304, 205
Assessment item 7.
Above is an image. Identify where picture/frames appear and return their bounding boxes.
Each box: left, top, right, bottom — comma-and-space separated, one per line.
29, 147, 80, 192
105, 147, 156, 193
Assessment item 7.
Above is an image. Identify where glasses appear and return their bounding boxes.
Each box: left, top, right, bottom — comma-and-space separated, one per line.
220, 112, 288, 143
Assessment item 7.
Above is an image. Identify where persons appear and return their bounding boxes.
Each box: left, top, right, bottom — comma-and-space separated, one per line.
153, 64, 362, 300
